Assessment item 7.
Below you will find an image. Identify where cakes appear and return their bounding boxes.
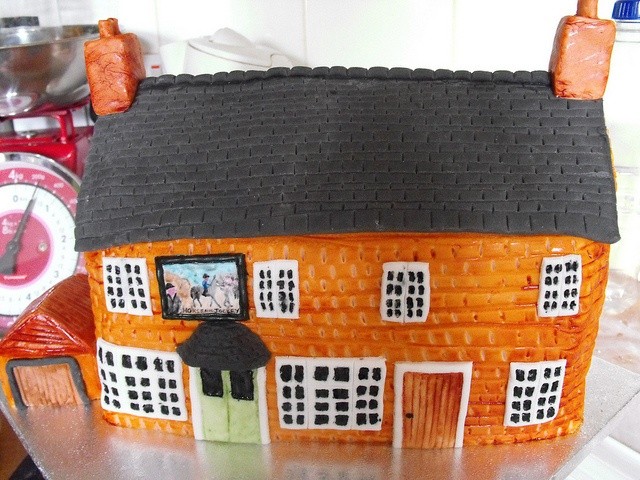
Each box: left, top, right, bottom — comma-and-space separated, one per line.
0, 0, 621, 449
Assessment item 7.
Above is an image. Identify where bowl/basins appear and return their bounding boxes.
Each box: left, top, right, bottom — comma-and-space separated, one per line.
0, 23, 102, 116
